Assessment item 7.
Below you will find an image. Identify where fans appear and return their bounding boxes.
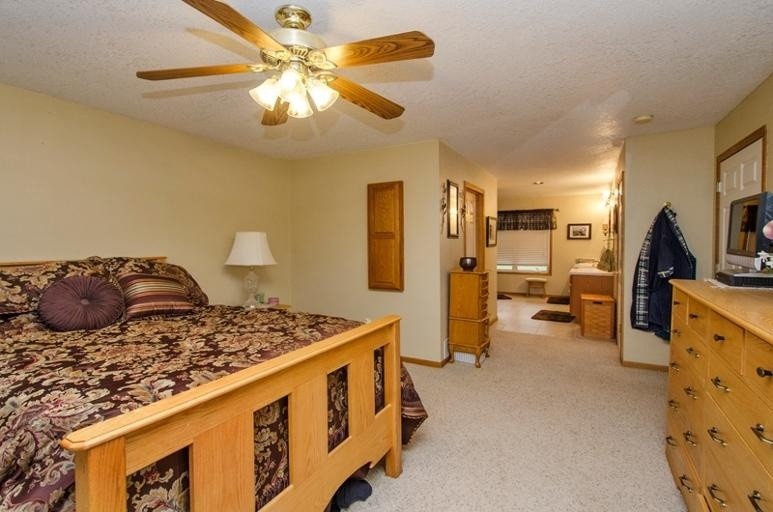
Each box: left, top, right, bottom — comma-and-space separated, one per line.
138, 0, 435, 126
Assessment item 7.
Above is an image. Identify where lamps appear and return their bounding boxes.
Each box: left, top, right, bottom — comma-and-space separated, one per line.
249, 65, 343, 120
224, 232, 277, 310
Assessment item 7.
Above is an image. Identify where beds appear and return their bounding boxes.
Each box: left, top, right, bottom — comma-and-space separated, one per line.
0, 256, 402, 512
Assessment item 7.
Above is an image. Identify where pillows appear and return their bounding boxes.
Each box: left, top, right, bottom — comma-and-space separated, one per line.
121, 273, 196, 318
40, 275, 122, 332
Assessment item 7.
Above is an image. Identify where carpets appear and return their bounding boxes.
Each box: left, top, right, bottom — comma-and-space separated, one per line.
547, 297, 570, 304
497, 294, 512, 299
531, 310, 575, 322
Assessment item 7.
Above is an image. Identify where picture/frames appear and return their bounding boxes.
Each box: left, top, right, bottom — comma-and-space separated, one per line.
487, 217, 497, 247
447, 179, 459, 238
568, 224, 591, 239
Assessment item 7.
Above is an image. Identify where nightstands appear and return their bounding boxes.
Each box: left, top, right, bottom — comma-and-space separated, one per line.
242, 302, 291, 311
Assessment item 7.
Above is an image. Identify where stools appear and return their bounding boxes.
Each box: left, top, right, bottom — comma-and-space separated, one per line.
526, 278, 547, 297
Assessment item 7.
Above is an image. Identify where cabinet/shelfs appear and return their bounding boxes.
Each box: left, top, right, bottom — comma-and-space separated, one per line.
569, 263, 616, 321
664, 278, 773, 511
580, 294, 614, 339
448, 270, 491, 367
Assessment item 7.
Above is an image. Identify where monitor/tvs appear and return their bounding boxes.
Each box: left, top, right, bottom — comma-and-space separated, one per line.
724, 191, 773, 277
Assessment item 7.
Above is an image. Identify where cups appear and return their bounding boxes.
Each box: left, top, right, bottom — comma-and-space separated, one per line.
255, 294, 264, 303
269, 297, 279, 305
